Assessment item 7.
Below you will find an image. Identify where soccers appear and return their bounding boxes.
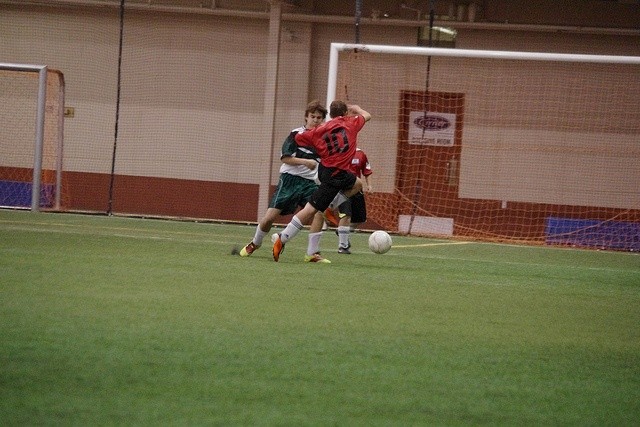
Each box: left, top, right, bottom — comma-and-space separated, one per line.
368, 230, 392, 254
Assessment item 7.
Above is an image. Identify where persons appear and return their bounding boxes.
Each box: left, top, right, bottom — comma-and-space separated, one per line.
271, 101, 372, 262
338, 148, 373, 254
239, 101, 331, 264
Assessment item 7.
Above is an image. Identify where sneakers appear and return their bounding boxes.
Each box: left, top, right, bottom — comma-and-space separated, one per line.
325, 208, 339, 227
240, 240, 260, 256
338, 244, 352, 254
305, 253, 330, 263
271, 232, 285, 262
